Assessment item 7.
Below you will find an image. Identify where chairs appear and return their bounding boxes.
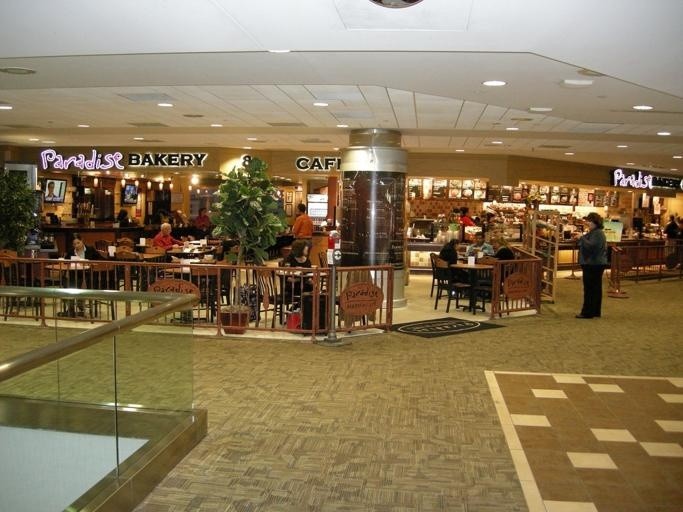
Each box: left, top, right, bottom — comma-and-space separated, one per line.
253, 260, 380, 333
427, 240, 520, 318
0, 233, 254, 326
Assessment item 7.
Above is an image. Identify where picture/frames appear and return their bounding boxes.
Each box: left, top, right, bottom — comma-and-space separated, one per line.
284, 204, 292, 218
285, 191, 292, 203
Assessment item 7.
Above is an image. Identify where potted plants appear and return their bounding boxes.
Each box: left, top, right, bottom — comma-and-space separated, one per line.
208, 157, 288, 334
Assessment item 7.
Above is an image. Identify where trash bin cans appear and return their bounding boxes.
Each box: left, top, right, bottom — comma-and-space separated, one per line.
303, 292, 329, 336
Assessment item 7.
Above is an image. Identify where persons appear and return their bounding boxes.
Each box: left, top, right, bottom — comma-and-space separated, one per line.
153, 223, 184, 250
129, 188, 135, 201
662, 214, 678, 238
55, 233, 104, 316
481, 238, 515, 278
194, 207, 211, 230
170, 210, 184, 228
291, 203, 315, 251
466, 231, 493, 257
47, 182, 60, 200
282, 239, 315, 300
459, 207, 478, 226
436, 239, 467, 284
674, 218, 683, 279
569, 213, 608, 318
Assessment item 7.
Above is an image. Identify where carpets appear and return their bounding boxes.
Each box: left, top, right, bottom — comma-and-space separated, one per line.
381, 315, 507, 340
478, 363, 683, 512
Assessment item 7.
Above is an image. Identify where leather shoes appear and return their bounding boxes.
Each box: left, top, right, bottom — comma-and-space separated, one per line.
575, 313, 593, 318
592, 314, 600, 317
